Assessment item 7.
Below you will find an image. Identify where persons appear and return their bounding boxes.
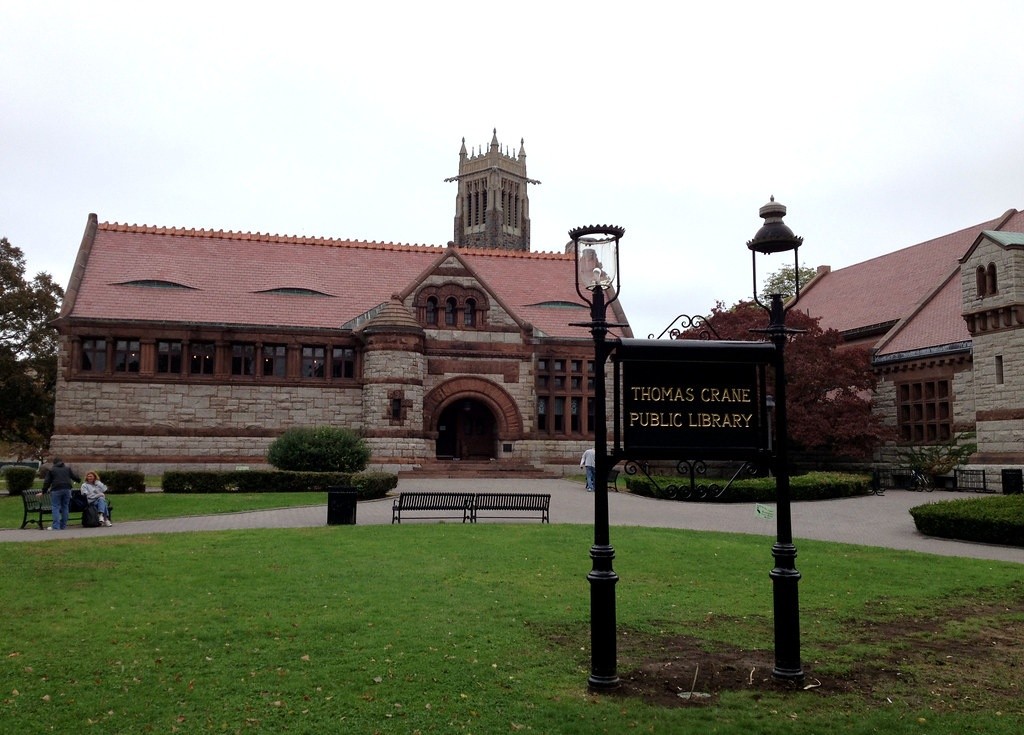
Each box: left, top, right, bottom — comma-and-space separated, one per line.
35, 457, 81, 531
580, 446, 595, 492
81, 471, 112, 527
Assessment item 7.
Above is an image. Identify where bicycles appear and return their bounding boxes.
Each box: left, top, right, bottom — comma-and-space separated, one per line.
902, 465, 936, 493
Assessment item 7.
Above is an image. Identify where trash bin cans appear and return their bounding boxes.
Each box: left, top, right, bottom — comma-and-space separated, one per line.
1001, 468, 1022, 495
326, 484, 359, 526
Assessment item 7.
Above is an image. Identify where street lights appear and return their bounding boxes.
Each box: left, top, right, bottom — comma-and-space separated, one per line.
568, 224, 628, 693
746, 194, 804, 692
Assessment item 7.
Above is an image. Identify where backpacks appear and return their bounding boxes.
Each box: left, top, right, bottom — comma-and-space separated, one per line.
81, 502, 102, 528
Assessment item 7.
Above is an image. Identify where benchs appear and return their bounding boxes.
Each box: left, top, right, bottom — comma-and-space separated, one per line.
18, 490, 113, 530
392, 492, 551, 524
585, 470, 621, 492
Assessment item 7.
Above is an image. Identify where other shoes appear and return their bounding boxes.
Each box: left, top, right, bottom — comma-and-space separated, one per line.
587, 488, 592, 492
97, 513, 104, 522
101, 519, 112, 527
47, 527, 59, 531
